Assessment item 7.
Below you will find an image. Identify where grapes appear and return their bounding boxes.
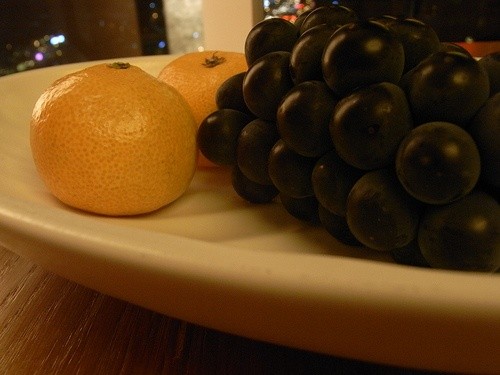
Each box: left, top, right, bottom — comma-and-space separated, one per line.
197, 3, 500, 277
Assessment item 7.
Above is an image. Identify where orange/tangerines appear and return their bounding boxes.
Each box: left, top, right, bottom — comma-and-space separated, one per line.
30, 52, 247, 216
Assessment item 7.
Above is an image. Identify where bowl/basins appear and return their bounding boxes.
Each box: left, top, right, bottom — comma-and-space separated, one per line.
0, 54, 500, 375
